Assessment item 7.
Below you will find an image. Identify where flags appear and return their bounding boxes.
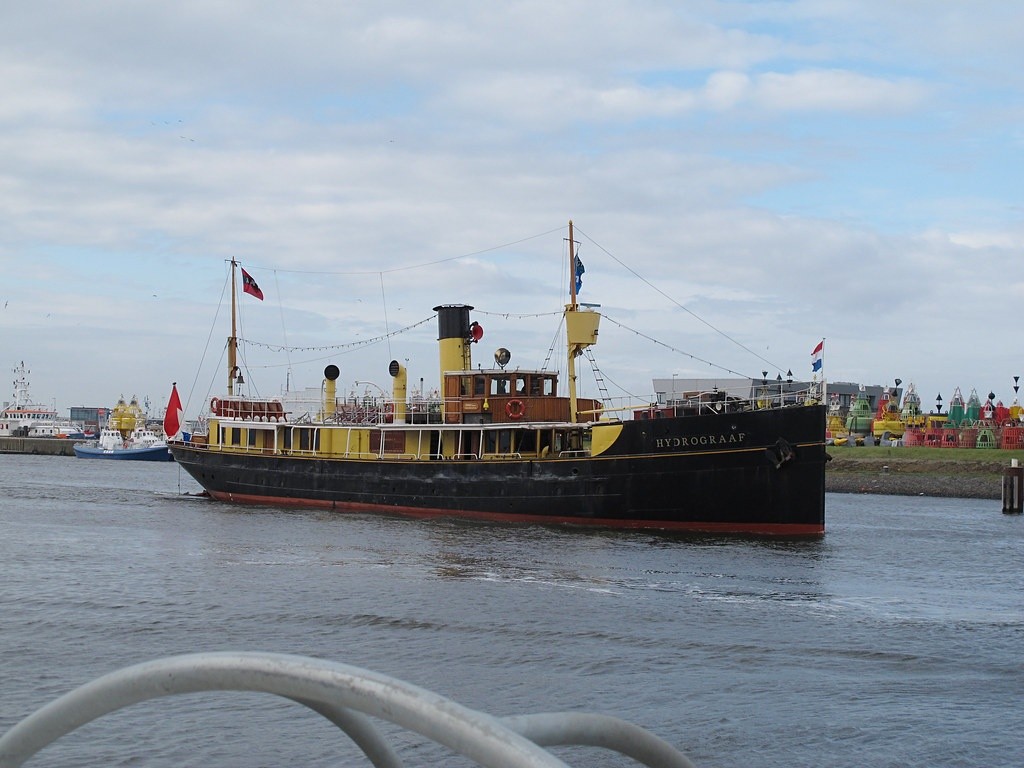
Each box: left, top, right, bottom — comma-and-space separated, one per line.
811, 341, 822, 372
241, 268, 263, 300
570, 255, 585, 296
163, 385, 183, 437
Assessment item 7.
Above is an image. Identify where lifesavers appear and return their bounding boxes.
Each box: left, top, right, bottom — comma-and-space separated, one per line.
383, 401, 394, 418
210, 397, 220, 412
505, 398, 526, 420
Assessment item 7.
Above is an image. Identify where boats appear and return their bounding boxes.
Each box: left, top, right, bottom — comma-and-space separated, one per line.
0, 360, 100, 439
73, 420, 191, 464
163, 220, 829, 545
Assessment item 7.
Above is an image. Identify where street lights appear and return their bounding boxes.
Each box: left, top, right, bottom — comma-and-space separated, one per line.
672, 373, 678, 401
51, 398, 55, 437
